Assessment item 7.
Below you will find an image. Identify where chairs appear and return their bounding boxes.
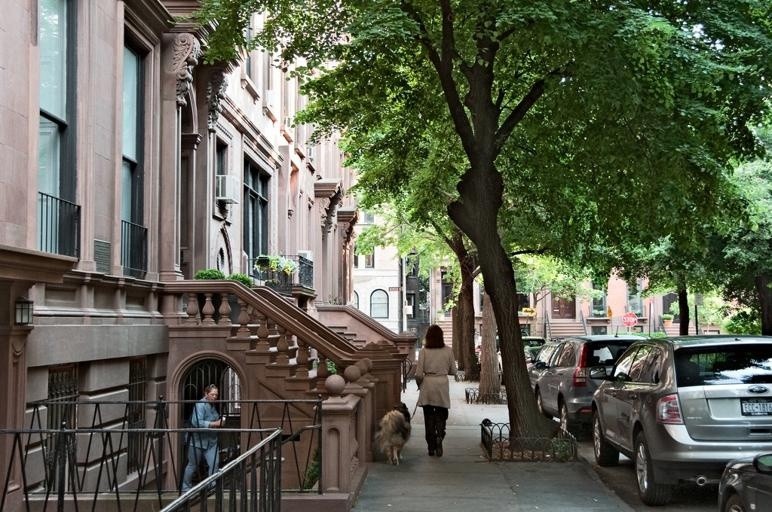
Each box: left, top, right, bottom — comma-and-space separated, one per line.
674, 360, 700, 386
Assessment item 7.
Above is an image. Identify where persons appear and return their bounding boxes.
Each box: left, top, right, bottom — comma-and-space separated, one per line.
414, 324, 458, 459
183, 383, 227, 492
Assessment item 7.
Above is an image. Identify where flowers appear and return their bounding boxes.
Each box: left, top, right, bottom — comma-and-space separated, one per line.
256, 255, 297, 274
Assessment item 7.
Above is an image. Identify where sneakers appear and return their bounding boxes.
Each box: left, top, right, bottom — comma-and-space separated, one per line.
427, 437, 443, 457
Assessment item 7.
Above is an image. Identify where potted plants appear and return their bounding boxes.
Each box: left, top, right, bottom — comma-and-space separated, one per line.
195, 270, 252, 324
662, 314, 673, 326
265, 280, 281, 292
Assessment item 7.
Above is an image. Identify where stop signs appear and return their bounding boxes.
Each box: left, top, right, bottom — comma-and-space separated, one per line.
623, 313, 637, 327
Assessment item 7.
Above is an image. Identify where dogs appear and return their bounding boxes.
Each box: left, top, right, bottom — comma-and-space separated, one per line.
372, 400, 412, 467
